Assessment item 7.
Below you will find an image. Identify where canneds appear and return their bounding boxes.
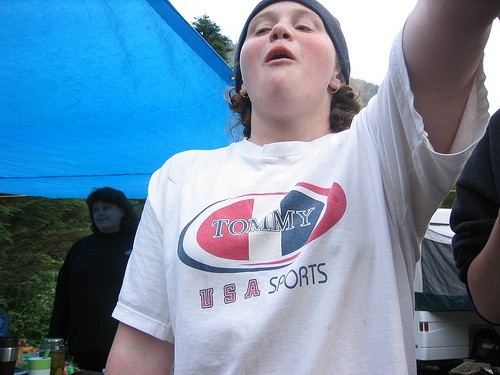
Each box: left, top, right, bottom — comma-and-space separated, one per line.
39, 338, 65, 375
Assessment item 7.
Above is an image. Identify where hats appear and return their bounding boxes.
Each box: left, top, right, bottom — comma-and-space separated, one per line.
233, 0, 350, 95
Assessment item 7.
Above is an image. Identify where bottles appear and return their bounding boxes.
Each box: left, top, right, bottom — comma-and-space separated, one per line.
39, 338, 64, 375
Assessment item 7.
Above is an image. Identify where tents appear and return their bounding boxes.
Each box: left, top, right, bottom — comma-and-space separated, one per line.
1, 1, 246, 199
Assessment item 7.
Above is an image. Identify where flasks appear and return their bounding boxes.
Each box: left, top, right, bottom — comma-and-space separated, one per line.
0, 336, 18, 375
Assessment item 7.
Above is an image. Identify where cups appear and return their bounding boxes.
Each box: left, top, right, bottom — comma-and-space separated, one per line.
28, 356, 52, 375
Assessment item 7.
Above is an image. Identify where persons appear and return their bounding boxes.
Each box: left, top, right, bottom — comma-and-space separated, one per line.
103, 0, 500, 375
449, 107, 500, 327
48, 186, 140, 372
